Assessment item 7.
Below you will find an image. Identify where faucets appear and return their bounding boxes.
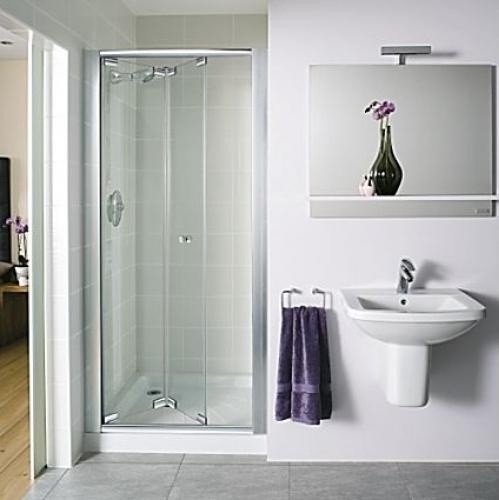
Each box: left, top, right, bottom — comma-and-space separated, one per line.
395, 259, 415, 294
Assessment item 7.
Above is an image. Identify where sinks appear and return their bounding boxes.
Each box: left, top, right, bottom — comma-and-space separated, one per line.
339, 287, 487, 347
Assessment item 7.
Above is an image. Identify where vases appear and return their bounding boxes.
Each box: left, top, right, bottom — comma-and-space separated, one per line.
14, 265, 29, 288
370, 123, 404, 196
366, 131, 385, 180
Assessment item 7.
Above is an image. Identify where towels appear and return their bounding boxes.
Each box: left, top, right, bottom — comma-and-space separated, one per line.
273, 306, 332, 426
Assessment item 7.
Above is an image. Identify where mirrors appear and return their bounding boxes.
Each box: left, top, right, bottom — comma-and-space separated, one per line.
102, 50, 257, 428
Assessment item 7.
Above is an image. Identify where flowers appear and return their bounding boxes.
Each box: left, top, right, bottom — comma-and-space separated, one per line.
4, 215, 30, 264
364, 98, 388, 152
371, 99, 403, 190
